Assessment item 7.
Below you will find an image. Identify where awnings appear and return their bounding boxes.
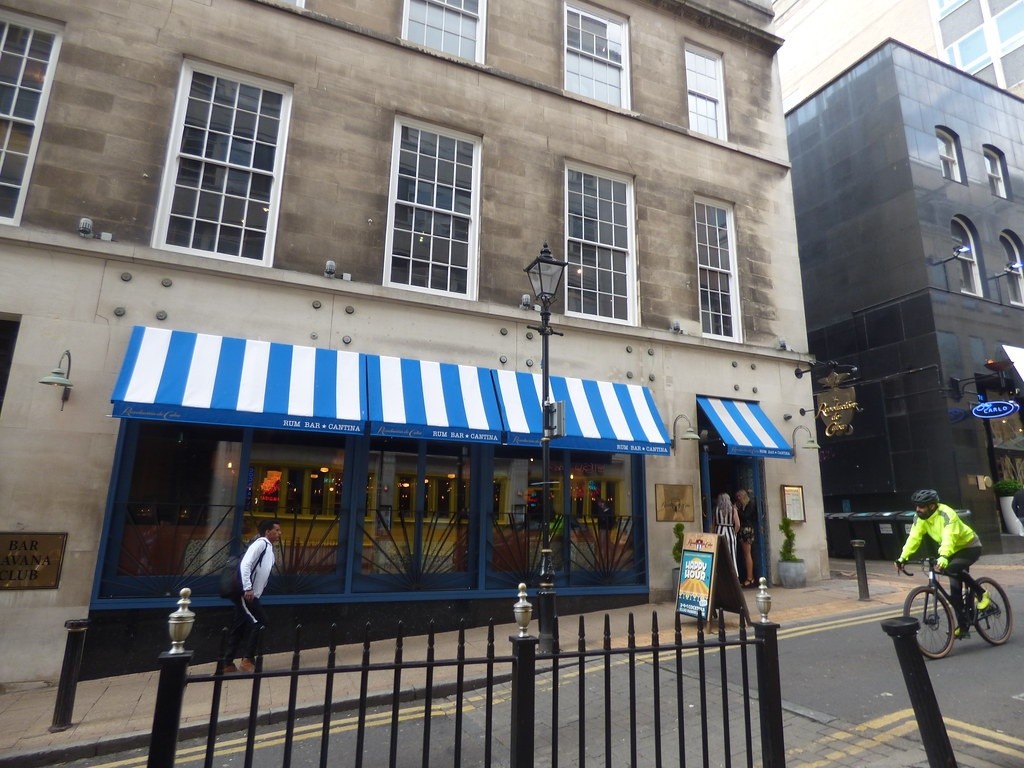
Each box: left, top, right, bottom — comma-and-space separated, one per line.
110, 325, 368, 436
491, 367, 673, 457
367, 352, 504, 447
696, 396, 793, 459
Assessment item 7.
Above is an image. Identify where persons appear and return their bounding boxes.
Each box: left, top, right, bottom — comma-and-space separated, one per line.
595, 501, 616, 569
892, 488, 990, 636
735, 489, 757, 587
712, 492, 740, 579
1011, 491, 1024, 528
217, 519, 282, 674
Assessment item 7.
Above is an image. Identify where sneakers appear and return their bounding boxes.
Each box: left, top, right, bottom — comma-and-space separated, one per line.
954, 626, 970, 639
977, 590, 992, 611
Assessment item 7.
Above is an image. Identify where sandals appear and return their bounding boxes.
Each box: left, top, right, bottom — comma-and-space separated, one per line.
742, 578, 755, 588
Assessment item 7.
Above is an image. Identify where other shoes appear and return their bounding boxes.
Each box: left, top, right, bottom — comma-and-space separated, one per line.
224, 662, 240, 673
238, 657, 255, 673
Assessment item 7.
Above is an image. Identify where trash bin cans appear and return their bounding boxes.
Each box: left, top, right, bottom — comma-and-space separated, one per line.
824, 510, 972, 564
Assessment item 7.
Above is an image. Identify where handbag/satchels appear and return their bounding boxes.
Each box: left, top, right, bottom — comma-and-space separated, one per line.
219, 566, 236, 599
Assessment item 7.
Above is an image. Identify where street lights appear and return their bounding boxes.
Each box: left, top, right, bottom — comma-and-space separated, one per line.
523, 239, 569, 654
974, 371, 1015, 484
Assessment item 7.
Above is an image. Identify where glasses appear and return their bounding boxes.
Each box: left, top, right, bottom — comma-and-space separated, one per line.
913, 504, 928, 508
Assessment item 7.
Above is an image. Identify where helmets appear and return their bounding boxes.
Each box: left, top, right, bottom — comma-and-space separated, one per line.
911, 488, 938, 505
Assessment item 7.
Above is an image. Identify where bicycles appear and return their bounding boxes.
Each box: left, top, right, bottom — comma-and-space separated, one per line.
895, 556, 1013, 659
